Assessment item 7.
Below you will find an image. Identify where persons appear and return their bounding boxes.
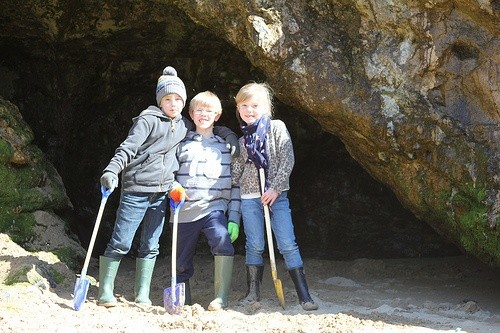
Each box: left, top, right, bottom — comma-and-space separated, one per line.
229, 81, 319, 312
170, 91, 240, 311
98, 66, 241, 307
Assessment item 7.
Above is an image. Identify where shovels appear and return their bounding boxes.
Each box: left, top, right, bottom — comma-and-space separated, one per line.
74, 178, 117, 310
258, 168, 285, 306
163, 193, 187, 316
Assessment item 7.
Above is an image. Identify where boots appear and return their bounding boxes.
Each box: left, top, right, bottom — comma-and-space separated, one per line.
287, 267, 319, 310
208, 255, 234, 310
134, 258, 156, 305
98, 254, 122, 306
180, 280, 191, 305
238, 262, 265, 305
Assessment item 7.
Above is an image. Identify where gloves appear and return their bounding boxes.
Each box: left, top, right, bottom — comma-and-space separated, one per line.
170, 187, 189, 203
100, 171, 119, 189
227, 222, 239, 243
226, 133, 240, 157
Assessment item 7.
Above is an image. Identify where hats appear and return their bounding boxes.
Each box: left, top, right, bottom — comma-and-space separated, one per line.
156, 66, 186, 108
